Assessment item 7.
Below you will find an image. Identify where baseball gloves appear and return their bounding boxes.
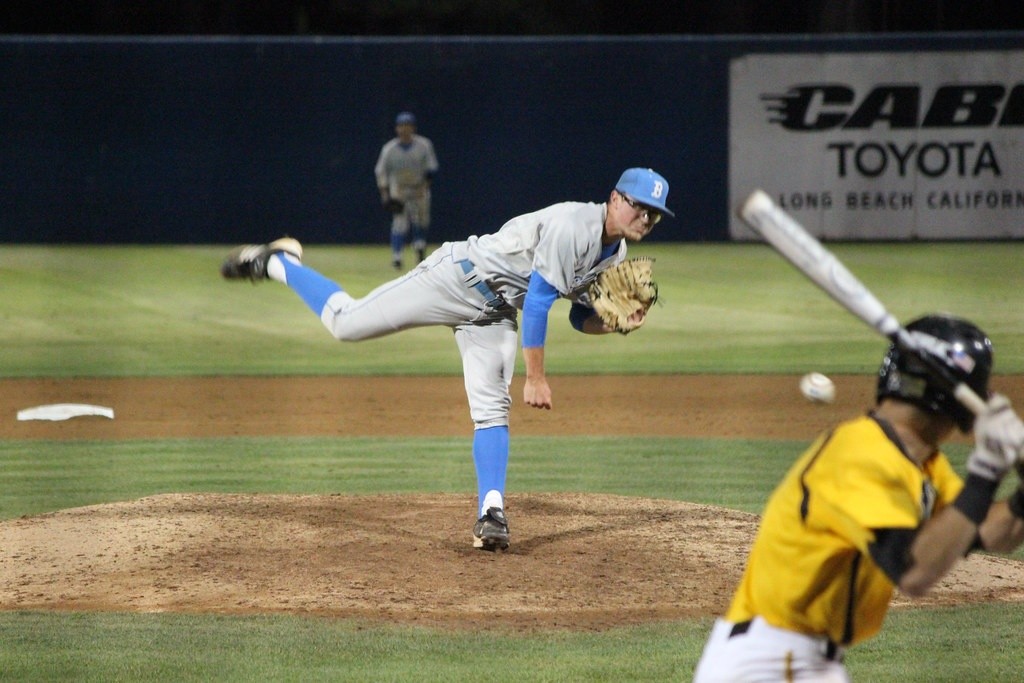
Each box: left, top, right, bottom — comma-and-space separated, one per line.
587, 256, 660, 335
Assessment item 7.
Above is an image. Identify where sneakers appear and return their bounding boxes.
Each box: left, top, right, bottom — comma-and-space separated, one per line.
474, 506, 510, 549
221, 236, 301, 285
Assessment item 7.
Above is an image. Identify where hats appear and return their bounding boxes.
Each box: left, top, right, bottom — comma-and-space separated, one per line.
397, 112, 414, 125
615, 167, 675, 218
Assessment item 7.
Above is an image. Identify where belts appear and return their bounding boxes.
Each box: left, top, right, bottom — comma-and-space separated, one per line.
730, 620, 837, 660
460, 260, 513, 311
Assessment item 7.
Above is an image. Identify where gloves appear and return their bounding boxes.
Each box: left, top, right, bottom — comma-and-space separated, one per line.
967, 393, 1024, 481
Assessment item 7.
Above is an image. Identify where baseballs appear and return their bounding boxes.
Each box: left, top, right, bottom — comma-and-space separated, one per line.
799, 372, 837, 406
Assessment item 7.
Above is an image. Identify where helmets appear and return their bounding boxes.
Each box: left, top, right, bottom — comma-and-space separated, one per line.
878, 316, 993, 432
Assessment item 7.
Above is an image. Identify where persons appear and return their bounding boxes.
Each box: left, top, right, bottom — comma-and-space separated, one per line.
693, 316, 1023, 683
220, 164, 670, 550
375, 111, 439, 268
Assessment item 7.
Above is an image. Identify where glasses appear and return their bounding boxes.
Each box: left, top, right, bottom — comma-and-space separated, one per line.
616, 190, 662, 224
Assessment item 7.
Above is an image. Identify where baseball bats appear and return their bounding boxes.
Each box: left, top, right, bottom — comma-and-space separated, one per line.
736, 187, 1024, 463
